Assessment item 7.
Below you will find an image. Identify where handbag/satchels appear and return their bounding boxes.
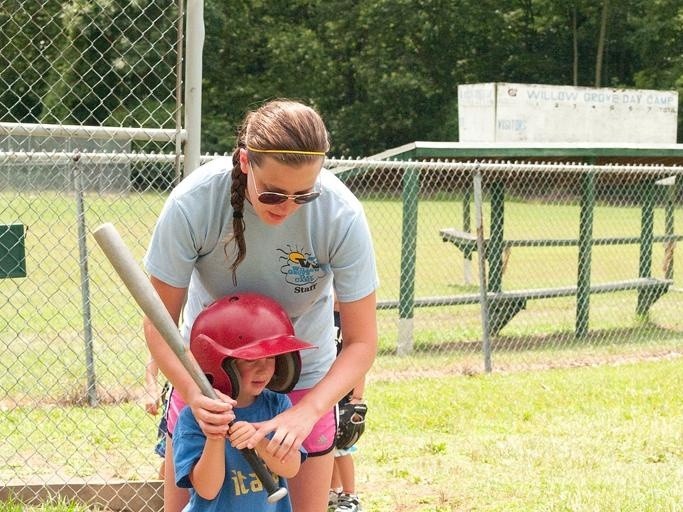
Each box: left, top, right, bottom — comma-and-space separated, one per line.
351, 396, 361, 400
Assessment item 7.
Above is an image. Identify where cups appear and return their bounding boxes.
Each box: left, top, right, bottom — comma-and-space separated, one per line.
248, 158, 323, 205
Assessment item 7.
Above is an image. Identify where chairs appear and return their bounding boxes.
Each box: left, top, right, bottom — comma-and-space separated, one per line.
328, 489, 345, 508
334, 493, 361, 512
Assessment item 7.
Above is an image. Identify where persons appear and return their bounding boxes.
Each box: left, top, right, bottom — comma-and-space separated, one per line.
324, 290, 366, 512
172, 351, 308, 512
143, 96, 379, 511
143, 325, 174, 479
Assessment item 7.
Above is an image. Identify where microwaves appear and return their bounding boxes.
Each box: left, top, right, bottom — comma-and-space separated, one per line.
92, 222, 286, 504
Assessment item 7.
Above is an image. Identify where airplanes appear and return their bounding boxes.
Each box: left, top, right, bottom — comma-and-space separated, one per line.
334, 405, 366, 450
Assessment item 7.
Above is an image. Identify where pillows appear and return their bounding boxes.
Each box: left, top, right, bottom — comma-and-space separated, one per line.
191, 293, 321, 401
